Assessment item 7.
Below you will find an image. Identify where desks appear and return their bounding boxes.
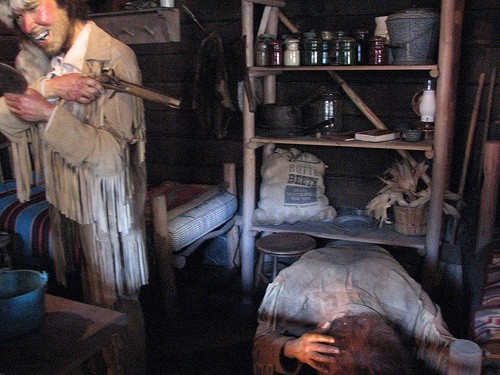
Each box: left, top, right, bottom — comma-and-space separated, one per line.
0, 295, 129, 375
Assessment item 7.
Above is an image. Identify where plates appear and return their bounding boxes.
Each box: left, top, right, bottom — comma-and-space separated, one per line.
331, 215, 380, 232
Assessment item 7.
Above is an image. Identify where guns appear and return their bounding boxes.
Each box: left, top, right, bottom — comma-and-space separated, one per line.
90, 66, 181, 111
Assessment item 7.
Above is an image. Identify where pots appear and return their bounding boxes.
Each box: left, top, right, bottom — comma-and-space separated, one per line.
0, 269, 49, 338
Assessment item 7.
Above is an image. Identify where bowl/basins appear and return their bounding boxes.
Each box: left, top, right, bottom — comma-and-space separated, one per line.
256, 103, 302, 129
405, 129, 421, 142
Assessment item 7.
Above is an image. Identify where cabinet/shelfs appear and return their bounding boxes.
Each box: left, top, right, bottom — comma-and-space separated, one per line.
239, 0, 463, 316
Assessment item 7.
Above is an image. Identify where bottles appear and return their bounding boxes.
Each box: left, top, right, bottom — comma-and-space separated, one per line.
316, 93, 342, 135
255, 29, 386, 67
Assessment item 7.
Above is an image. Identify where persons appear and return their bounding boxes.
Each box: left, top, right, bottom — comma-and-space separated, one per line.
0, 0, 149, 375
255, 241, 488, 375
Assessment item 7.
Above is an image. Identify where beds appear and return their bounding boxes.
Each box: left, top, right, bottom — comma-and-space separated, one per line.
1, 141, 240, 325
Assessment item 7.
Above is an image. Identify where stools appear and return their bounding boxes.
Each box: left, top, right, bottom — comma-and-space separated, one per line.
255, 232, 316, 289
